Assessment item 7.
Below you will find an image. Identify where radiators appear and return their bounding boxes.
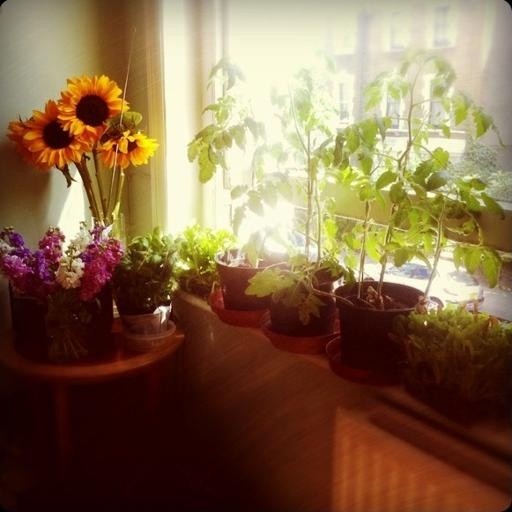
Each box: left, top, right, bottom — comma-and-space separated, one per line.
331, 409, 512, 508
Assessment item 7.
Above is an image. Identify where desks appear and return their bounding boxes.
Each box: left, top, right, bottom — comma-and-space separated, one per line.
10, 312, 187, 464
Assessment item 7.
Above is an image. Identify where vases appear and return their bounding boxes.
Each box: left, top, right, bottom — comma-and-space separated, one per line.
7, 294, 112, 345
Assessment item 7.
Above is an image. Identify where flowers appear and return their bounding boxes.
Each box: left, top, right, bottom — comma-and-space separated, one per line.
7, 72, 160, 236
0, 221, 123, 306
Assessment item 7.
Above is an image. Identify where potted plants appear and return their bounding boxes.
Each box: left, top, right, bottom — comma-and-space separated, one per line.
188, 60, 292, 310
392, 179, 510, 401
227, 70, 355, 339
108, 226, 175, 347
248, 45, 511, 371
175, 221, 233, 297
